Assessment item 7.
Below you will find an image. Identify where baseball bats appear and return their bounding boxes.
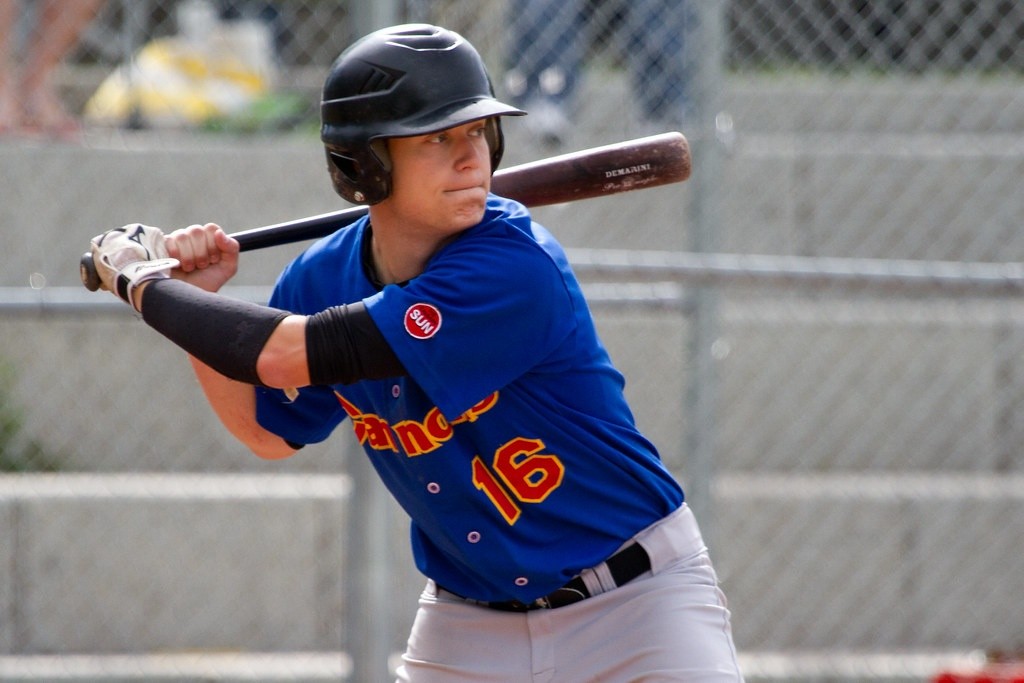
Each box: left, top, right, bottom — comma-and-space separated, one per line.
77, 131, 692, 293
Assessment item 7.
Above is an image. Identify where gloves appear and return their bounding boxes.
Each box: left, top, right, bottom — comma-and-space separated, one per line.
90, 223, 181, 314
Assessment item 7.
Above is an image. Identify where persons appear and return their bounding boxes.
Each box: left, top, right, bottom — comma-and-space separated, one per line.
503, 0, 698, 143
92, 22, 743, 682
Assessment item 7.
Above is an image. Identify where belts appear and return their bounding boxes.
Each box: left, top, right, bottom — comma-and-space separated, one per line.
436, 541, 650, 613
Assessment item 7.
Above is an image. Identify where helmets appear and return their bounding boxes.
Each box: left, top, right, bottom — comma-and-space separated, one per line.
320, 23, 530, 207
